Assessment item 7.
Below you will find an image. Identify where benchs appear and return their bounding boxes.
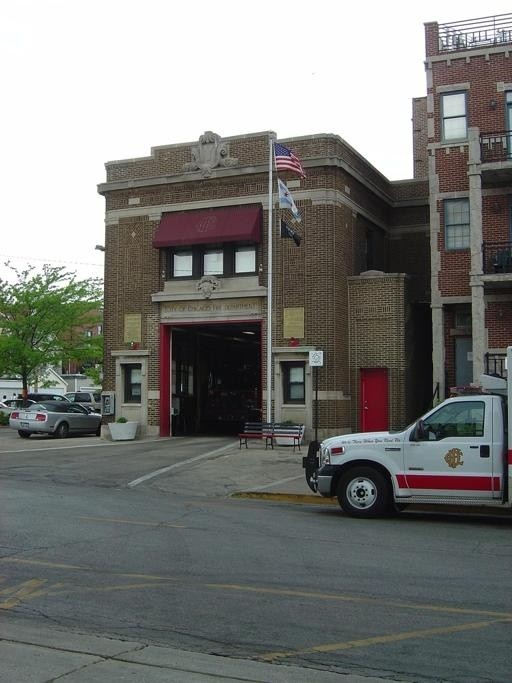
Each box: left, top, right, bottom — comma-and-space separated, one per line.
235, 418, 303, 452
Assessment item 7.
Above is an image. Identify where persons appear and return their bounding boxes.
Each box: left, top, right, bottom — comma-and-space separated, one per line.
2, 394, 8, 401
13, 392, 18, 406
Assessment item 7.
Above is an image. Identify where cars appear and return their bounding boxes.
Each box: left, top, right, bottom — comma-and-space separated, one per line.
0, 390, 102, 438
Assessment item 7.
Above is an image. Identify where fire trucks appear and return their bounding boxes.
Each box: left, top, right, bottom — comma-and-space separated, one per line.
206, 360, 259, 424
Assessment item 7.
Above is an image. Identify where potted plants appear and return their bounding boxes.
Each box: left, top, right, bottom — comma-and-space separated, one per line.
108, 414, 140, 441
273, 419, 307, 446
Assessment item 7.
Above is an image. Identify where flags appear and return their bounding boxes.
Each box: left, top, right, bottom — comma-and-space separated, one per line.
277, 177, 302, 224
279, 218, 302, 247
273, 142, 306, 176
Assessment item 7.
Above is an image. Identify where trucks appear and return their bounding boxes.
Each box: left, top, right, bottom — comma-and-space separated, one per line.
302, 345, 512, 518
